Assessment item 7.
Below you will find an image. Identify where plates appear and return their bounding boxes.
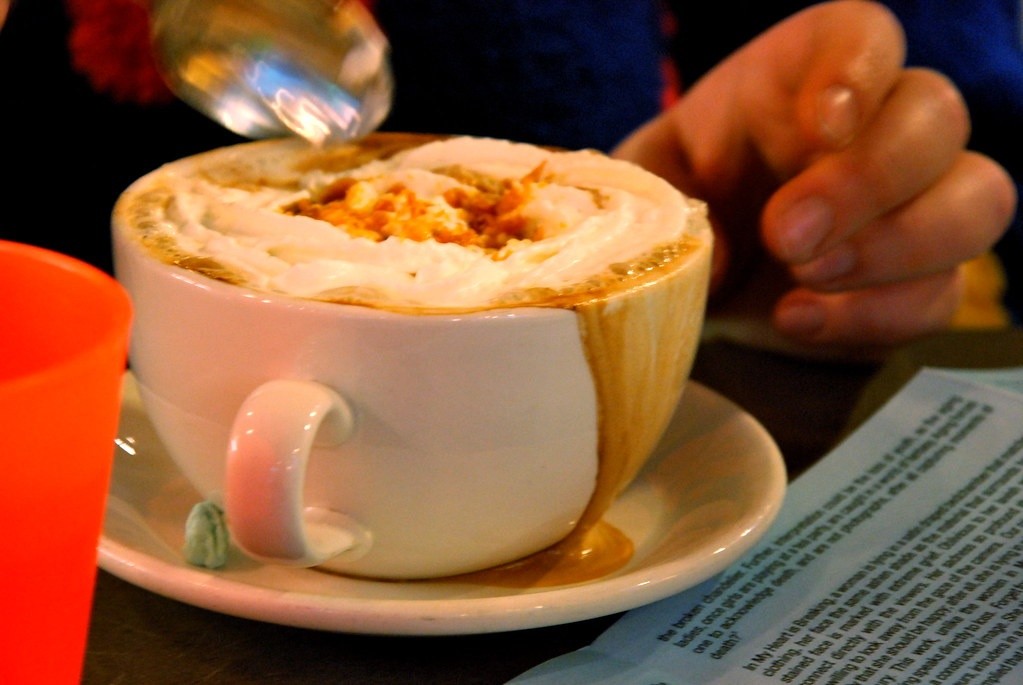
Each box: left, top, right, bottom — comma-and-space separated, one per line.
102, 370, 786, 636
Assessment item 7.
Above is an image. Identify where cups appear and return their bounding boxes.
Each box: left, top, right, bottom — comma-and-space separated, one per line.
113, 132, 713, 580
0, 239, 135, 684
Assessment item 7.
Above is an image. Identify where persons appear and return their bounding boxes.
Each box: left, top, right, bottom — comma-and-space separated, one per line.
0, 0, 1023, 349
